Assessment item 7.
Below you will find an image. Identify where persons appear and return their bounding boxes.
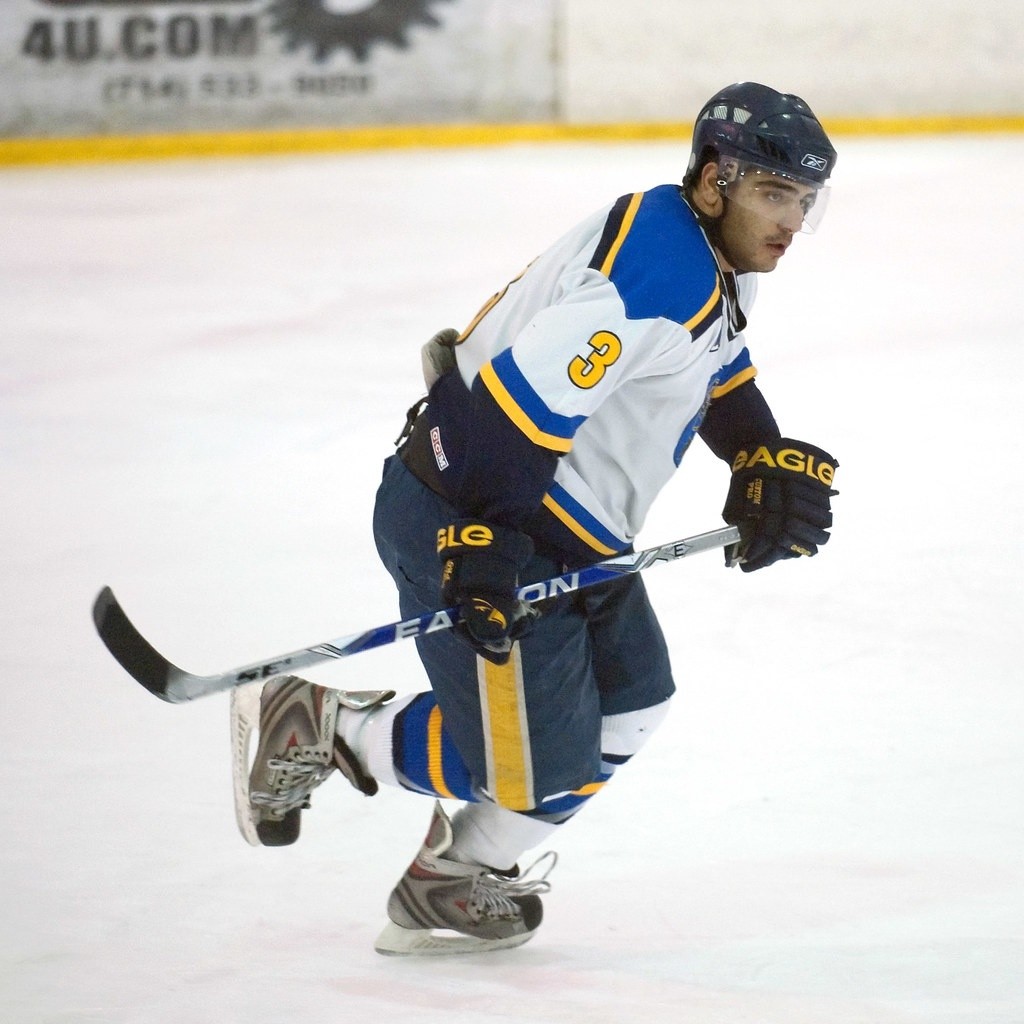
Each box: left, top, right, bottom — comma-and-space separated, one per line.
230, 82, 840, 956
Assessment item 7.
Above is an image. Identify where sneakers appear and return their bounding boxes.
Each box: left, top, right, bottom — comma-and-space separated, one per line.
229, 674, 396, 849
372, 800, 558, 957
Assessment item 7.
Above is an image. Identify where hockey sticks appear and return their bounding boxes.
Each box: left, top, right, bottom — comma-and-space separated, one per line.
93, 529, 742, 705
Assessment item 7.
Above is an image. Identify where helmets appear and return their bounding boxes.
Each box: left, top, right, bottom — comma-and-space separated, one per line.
683, 82, 838, 230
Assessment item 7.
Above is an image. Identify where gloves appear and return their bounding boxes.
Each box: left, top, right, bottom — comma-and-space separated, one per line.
723, 437, 840, 572
437, 519, 538, 667
